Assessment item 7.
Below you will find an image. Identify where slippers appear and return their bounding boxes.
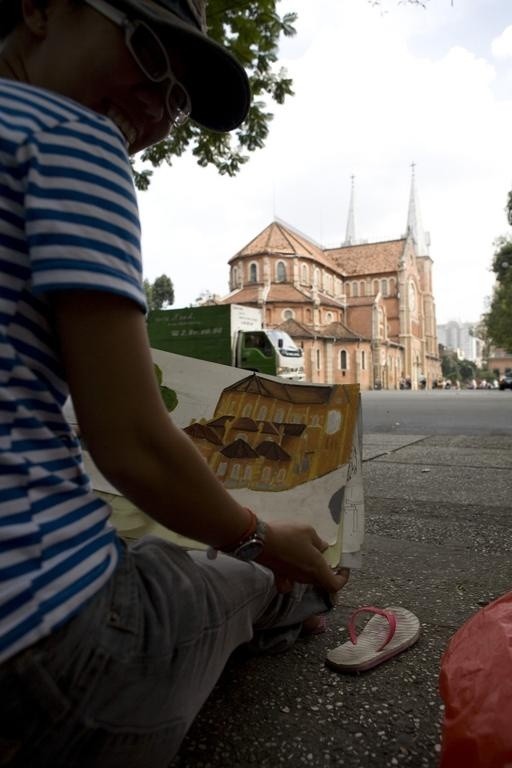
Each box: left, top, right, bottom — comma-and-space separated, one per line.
323, 602, 425, 674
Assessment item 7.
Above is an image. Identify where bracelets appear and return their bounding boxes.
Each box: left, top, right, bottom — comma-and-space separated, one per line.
213, 509, 258, 552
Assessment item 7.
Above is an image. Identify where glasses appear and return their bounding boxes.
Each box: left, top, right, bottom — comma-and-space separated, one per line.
84, 0, 193, 129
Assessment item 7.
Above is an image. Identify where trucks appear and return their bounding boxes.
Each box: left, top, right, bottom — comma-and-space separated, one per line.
144, 302, 310, 384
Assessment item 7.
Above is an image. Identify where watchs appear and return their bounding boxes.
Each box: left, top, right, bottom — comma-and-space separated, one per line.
224, 514, 267, 563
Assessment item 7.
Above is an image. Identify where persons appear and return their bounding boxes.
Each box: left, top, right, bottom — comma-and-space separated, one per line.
2, 3, 349, 768
248, 335, 256, 345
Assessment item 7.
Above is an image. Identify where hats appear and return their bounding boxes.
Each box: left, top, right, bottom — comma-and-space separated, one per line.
128, 0, 252, 134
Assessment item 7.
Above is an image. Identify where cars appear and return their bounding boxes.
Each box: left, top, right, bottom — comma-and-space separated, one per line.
400, 375, 512, 391
